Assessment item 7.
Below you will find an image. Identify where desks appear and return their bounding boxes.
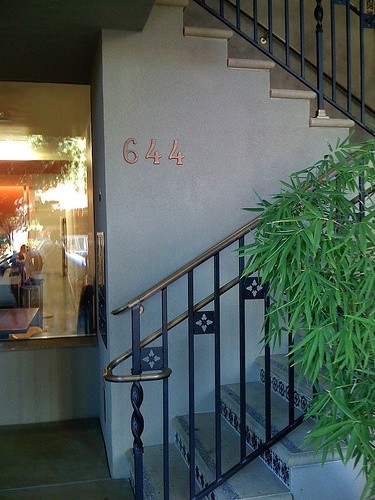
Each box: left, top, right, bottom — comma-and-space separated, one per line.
0, 306, 40, 333
